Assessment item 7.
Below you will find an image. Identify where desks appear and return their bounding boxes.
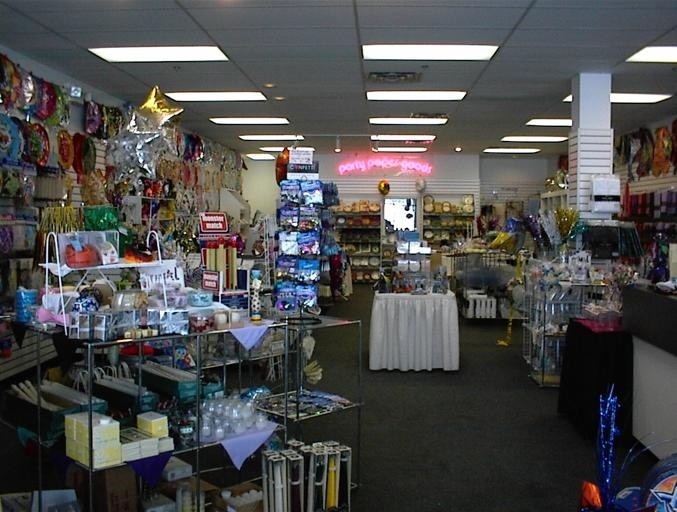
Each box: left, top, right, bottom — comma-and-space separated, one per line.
620, 283, 677, 463
369, 291, 460, 372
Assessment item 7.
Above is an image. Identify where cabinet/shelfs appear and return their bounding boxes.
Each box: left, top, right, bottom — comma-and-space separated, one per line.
523, 258, 622, 388
259, 181, 476, 314
444, 247, 529, 319
1, 229, 364, 509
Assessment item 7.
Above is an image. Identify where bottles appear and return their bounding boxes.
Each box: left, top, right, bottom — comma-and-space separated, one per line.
200, 387, 269, 441
